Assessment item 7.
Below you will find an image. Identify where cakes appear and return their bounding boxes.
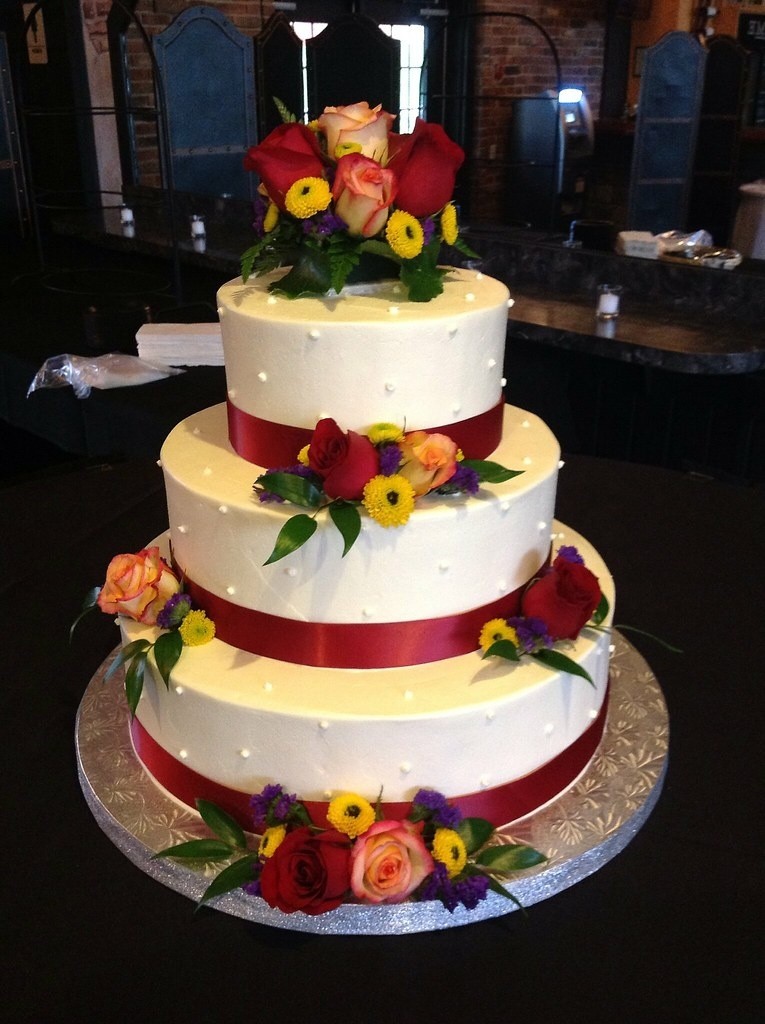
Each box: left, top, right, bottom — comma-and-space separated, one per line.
92, 260, 618, 861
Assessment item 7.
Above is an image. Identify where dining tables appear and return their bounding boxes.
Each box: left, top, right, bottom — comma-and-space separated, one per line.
0, 203, 765, 1024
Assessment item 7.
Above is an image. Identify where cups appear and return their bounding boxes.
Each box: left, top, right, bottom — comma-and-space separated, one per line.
120, 205, 135, 224
594, 285, 621, 320
190, 215, 206, 239
